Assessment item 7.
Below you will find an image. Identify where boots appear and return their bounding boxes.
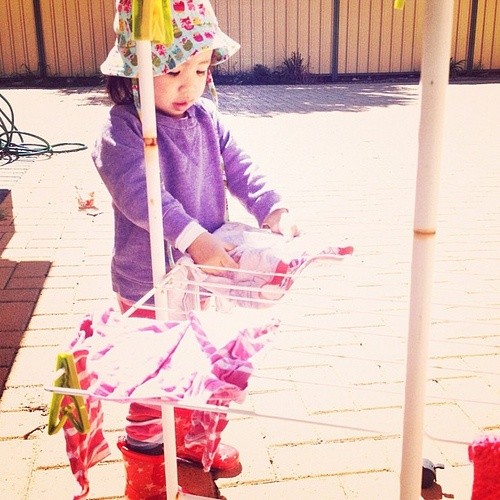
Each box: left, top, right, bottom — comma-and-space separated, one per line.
115, 437, 167, 500
176, 408, 240, 471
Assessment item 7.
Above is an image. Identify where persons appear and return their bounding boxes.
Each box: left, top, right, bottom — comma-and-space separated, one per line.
90, 0, 306, 500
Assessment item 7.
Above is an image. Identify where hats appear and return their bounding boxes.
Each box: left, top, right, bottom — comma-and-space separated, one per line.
100, 0, 243, 78
